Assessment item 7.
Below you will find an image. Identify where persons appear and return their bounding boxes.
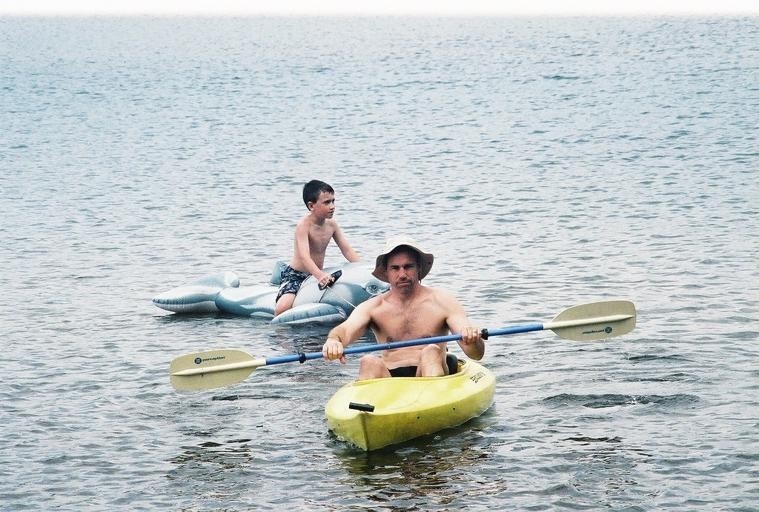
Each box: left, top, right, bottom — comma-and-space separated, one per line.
321, 241, 486, 379
274, 179, 361, 319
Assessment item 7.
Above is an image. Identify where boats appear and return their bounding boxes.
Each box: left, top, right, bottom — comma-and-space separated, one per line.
148, 261, 391, 330
323, 350, 499, 454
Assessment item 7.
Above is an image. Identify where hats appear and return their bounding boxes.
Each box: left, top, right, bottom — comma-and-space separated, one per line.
372, 237, 433, 282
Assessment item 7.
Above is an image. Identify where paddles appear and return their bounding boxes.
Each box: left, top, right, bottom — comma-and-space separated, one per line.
171, 299, 636, 392
